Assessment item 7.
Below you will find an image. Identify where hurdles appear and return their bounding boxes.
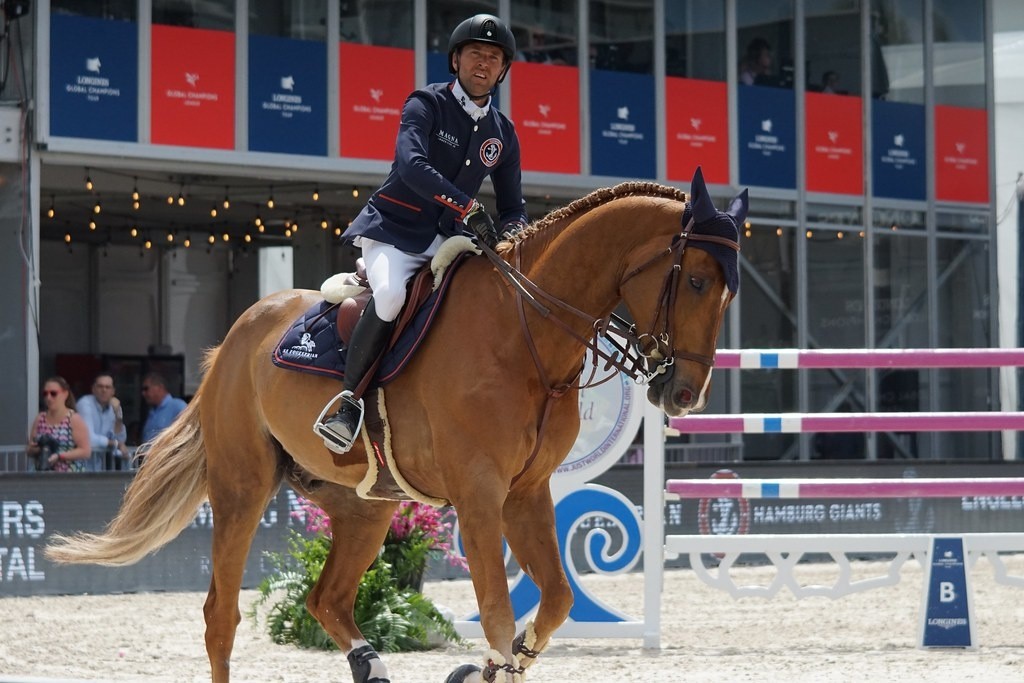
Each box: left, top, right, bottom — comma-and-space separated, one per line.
453, 319, 1024, 653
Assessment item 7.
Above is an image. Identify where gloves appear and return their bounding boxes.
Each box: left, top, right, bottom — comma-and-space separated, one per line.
466, 204, 498, 251
497, 222, 524, 241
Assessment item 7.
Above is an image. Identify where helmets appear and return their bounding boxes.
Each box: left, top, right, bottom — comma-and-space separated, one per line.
447, 14, 516, 77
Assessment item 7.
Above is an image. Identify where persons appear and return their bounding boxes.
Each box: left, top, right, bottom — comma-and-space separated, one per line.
26, 371, 190, 475
314, 12, 526, 454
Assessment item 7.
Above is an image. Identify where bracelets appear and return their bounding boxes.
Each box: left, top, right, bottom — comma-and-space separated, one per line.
56, 452, 61, 461
116, 417, 124, 423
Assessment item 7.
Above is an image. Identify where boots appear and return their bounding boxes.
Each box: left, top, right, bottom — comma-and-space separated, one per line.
322, 297, 399, 455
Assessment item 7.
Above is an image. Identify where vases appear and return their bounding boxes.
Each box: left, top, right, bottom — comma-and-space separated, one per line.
367, 539, 425, 594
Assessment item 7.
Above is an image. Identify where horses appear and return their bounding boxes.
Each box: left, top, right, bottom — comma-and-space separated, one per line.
42, 166, 749, 683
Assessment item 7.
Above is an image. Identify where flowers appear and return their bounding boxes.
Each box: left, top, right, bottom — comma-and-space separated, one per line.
241, 496, 478, 655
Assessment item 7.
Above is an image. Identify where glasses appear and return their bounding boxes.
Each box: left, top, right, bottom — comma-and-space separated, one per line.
43, 388, 66, 398
142, 386, 150, 391
